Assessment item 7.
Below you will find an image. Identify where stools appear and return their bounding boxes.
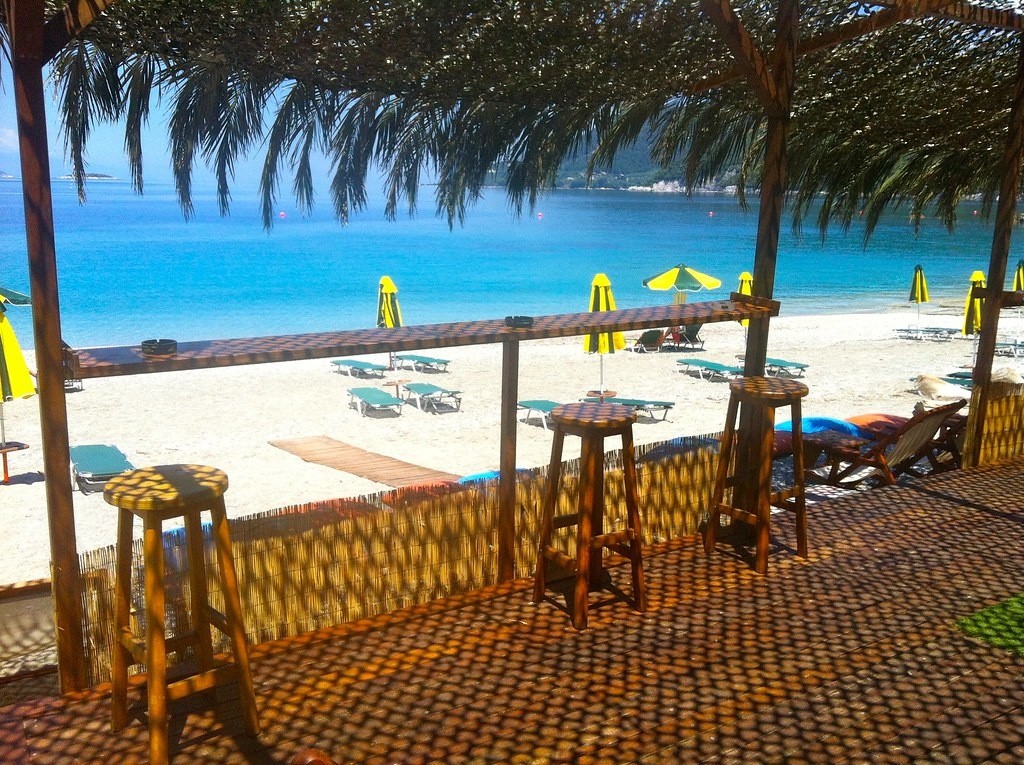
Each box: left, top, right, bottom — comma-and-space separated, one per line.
104, 463, 263, 765
531, 401, 650, 632
704, 377, 809, 575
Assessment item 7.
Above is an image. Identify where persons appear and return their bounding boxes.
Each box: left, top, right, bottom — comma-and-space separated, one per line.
663, 326, 681, 341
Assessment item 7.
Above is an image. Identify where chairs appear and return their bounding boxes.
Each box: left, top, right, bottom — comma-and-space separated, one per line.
67, 444, 135, 496
631, 323, 705, 353
676, 358, 744, 383
973, 337, 1024, 357
892, 323, 963, 345
831, 399, 967, 485
583, 397, 675, 420
392, 355, 453, 373
330, 359, 389, 379
346, 387, 407, 418
516, 400, 562, 429
402, 381, 465, 413
909, 372, 973, 389
737, 355, 809, 378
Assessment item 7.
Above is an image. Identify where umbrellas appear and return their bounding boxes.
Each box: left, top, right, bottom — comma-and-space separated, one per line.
963, 270, 987, 366
583, 273, 626, 394
737, 271, 754, 348
908, 264, 930, 323
377, 276, 402, 370
0, 287, 37, 448
642, 263, 722, 304
1013, 259, 1024, 291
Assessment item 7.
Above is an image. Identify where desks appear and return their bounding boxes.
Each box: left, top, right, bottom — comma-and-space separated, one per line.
0, 441, 29, 483
